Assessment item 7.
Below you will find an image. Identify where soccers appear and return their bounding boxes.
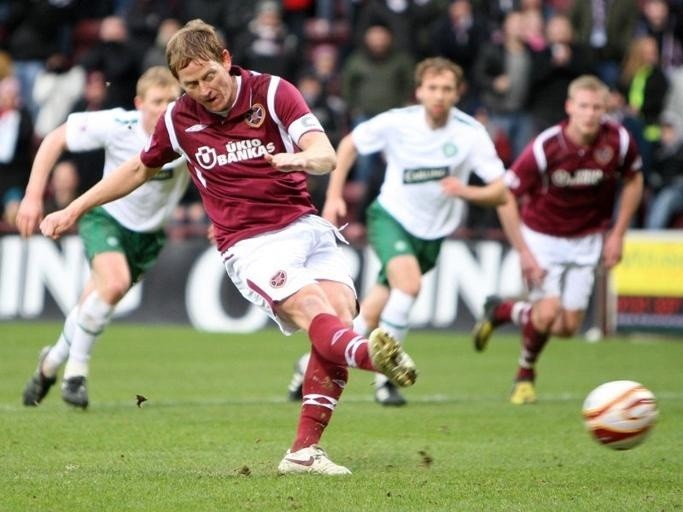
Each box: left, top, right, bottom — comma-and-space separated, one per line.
582, 380, 658, 450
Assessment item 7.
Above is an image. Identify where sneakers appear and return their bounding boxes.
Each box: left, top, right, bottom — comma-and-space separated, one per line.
279, 444, 352, 477
512, 380, 536, 404
22, 346, 56, 406
475, 294, 500, 349
368, 327, 418, 406
61, 376, 88, 407
289, 352, 310, 400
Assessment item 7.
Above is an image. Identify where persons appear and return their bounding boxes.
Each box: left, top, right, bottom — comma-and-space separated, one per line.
473, 76, 645, 405
287, 55, 509, 407
36, 18, 418, 475
15, 64, 192, 409
